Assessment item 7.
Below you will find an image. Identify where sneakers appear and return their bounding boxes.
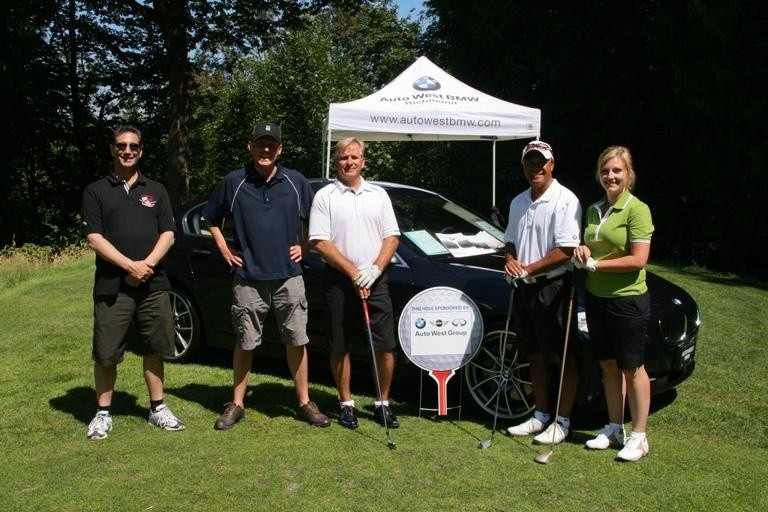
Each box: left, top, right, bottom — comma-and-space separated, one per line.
534, 420, 570, 446
148, 402, 187, 431
615, 436, 649, 462
87, 410, 113, 440
507, 417, 545, 438
586, 426, 622, 450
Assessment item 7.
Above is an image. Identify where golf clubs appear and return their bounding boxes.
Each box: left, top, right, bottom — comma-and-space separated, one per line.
359, 289, 396, 447
480, 286, 514, 449
534, 266, 578, 464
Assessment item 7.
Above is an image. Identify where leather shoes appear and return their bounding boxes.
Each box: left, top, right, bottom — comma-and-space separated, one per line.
375, 404, 399, 429
339, 404, 359, 428
215, 403, 244, 430
297, 399, 332, 427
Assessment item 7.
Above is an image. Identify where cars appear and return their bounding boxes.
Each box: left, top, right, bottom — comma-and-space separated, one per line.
123, 179, 699, 425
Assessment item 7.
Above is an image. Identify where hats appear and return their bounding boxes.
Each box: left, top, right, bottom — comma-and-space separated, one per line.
522, 140, 554, 166
252, 122, 282, 142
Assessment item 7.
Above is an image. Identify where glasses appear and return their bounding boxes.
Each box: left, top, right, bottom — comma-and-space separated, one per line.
114, 142, 143, 151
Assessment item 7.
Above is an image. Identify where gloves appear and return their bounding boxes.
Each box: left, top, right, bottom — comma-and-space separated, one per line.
355, 262, 380, 290
570, 256, 597, 272
504, 264, 530, 286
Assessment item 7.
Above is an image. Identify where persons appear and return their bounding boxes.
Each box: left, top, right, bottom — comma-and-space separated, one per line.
199, 122, 331, 430
571, 146, 655, 462
308, 137, 401, 428
503, 141, 583, 446
82, 126, 186, 440
